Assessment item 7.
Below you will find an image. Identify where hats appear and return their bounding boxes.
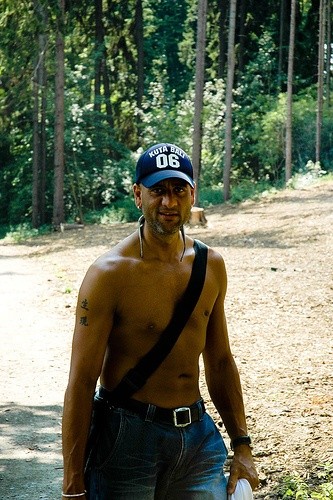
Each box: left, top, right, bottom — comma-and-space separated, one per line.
136, 143, 195, 189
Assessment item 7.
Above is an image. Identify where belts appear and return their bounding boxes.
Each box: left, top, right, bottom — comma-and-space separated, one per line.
96, 386, 207, 428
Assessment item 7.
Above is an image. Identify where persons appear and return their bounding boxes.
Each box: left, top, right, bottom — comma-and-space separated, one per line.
61, 145, 261, 499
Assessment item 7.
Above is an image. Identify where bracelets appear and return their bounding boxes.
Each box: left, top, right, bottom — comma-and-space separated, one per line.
230, 435, 252, 451
61, 489, 86, 497
